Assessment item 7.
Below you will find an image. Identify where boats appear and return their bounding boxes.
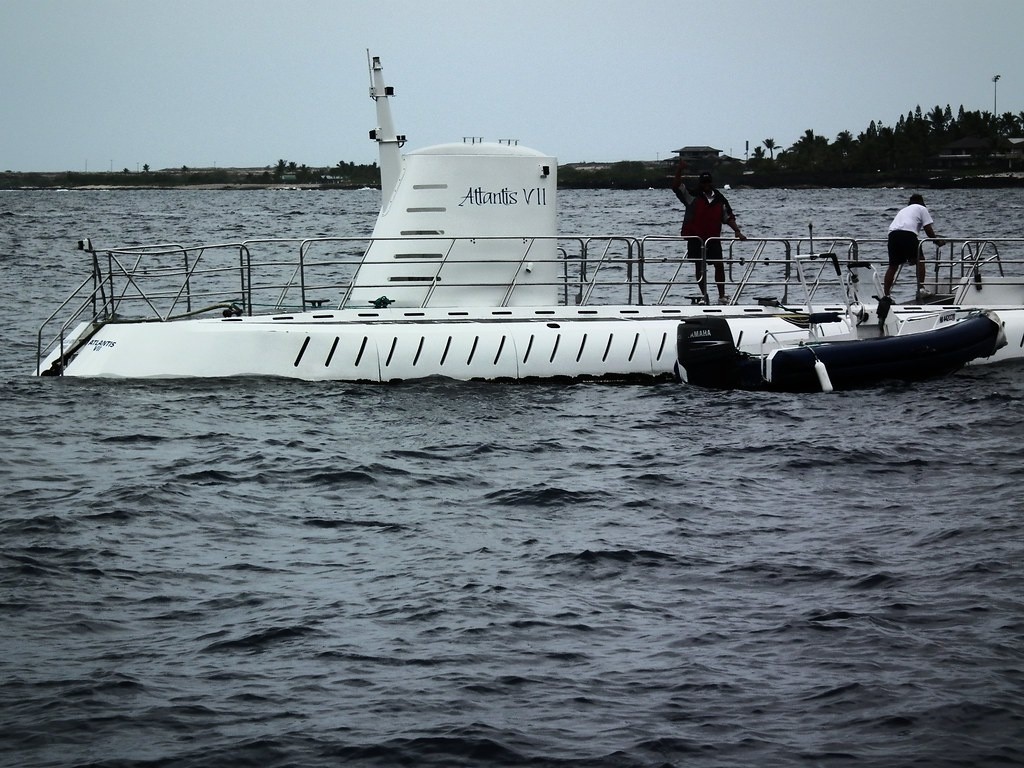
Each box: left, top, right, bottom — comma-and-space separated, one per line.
27, 47, 1024, 392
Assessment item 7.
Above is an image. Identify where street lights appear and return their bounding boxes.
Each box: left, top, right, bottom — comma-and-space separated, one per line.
993, 74, 1001, 117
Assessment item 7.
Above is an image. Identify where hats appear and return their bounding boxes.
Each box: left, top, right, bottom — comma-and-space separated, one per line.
908, 194, 926, 207
700, 172, 713, 184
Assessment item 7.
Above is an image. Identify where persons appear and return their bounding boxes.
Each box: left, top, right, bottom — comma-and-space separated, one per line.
884, 193, 947, 302
672, 169, 746, 305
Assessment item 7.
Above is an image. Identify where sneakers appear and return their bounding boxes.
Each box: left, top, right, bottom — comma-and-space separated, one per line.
698, 297, 709, 304
718, 297, 738, 305
916, 288, 935, 294
